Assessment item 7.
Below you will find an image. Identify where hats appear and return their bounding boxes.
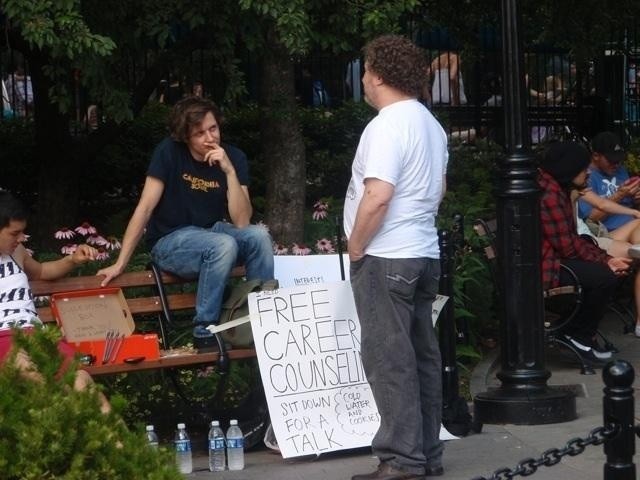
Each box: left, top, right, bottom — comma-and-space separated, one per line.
592, 131, 626, 162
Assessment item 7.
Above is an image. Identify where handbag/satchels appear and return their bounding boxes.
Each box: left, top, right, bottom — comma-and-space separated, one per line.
218, 279, 279, 348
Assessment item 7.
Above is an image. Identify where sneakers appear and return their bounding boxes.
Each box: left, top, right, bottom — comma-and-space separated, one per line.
562, 340, 616, 369
628, 243, 640, 259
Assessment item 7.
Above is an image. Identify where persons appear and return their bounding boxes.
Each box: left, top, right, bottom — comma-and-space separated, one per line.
1, 65, 36, 125
160, 77, 186, 104
1, 193, 127, 450
427, 38, 467, 107
98, 97, 274, 342
342, 36, 450, 480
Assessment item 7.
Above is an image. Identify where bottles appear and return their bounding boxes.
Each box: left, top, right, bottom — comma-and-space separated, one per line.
207, 420, 226, 471
175, 421, 192, 474
226, 420, 246, 471
144, 425, 159, 450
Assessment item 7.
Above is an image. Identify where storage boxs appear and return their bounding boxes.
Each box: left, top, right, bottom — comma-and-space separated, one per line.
48, 285, 162, 368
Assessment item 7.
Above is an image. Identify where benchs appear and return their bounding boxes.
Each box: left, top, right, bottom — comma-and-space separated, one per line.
474, 215, 635, 374
28, 264, 270, 441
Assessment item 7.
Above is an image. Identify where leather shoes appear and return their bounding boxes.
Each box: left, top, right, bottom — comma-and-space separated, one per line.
426, 458, 443, 476
352, 460, 426, 480
192, 336, 216, 348
